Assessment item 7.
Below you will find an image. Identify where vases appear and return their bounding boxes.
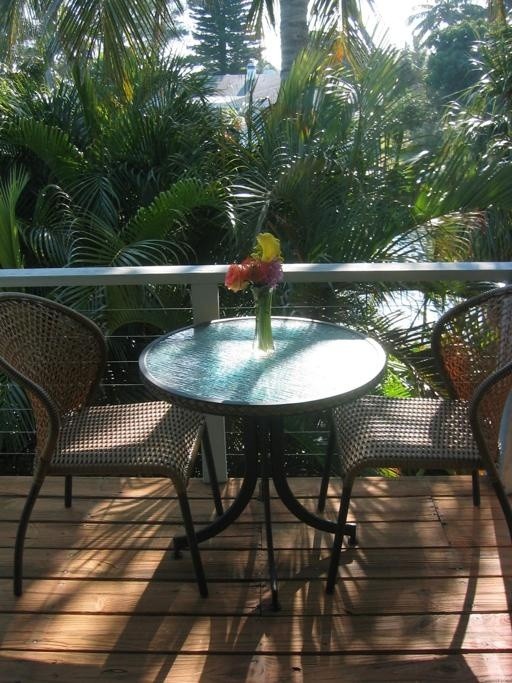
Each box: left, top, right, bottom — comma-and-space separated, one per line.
252, 289, 276, 356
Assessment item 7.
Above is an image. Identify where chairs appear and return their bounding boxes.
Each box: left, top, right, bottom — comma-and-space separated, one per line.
0, 290, 223, 600
317, 285, 512, 596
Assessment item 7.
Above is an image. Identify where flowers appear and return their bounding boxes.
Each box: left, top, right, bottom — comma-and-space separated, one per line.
222, 231, 284, 351
